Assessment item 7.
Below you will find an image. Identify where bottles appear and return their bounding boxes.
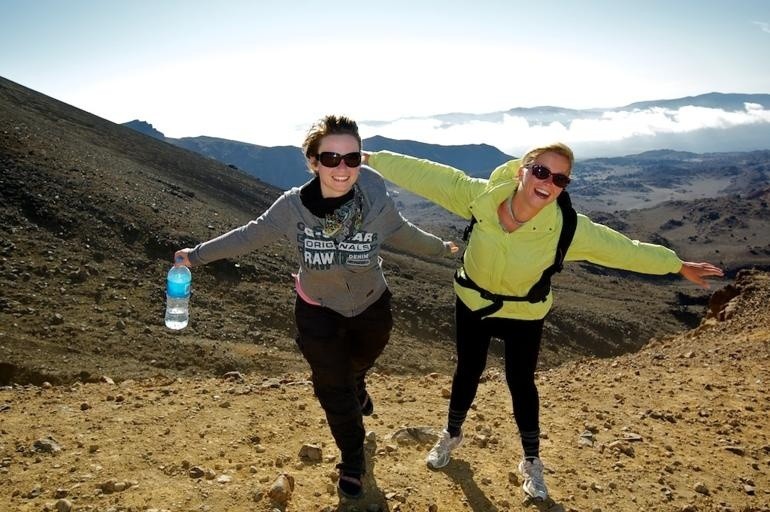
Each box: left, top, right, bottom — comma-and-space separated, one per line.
165, 256, 192, 330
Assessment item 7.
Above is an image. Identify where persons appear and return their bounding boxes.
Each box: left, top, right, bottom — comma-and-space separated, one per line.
360, 143, 727, 502
173, 118, 461, 499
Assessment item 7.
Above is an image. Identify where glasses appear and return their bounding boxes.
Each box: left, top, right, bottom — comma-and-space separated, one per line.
525, 164, 572, 189
315, 151, 362, 168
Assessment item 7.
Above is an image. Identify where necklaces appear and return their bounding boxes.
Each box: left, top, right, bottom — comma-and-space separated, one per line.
507, 190, 524, 225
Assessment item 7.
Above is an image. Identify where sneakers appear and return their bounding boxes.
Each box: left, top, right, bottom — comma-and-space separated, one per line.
338, 455, 366, 499
424, 425, 465, 469
518, 457, 547, 502
358, 389, 374, 417
